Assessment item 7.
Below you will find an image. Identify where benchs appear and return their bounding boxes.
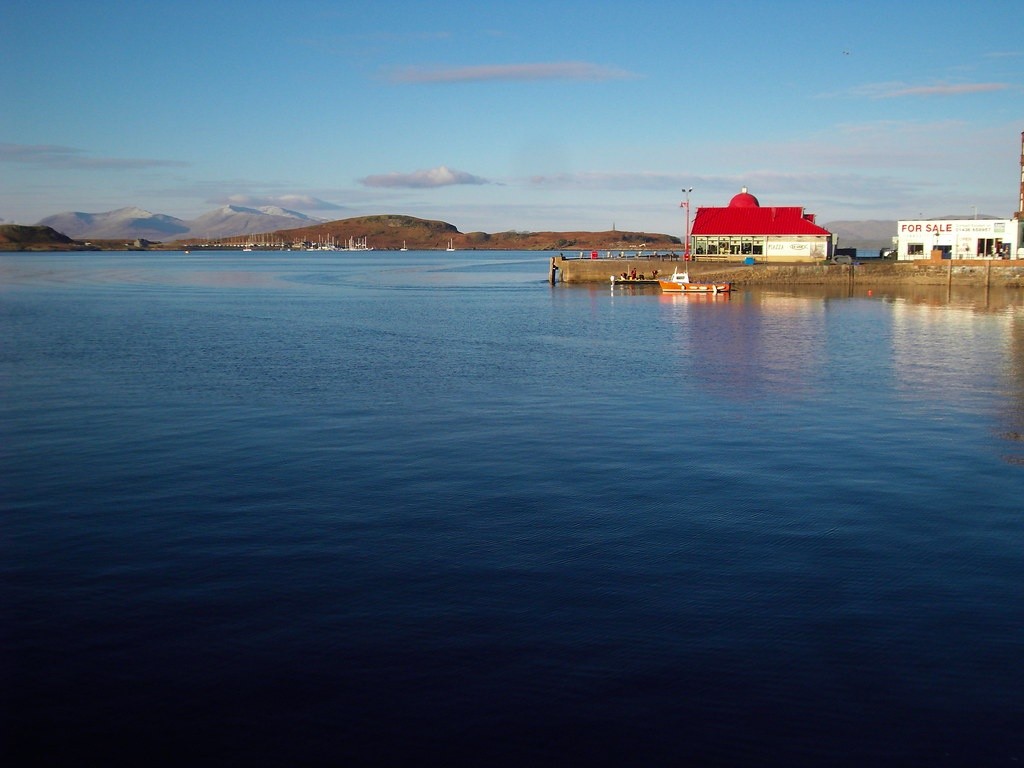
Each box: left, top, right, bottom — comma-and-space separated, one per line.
646, 255, 660, 260
662, 255, 679, 261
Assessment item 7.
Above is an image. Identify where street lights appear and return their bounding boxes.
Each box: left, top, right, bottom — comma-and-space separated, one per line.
681, 187, 693, 260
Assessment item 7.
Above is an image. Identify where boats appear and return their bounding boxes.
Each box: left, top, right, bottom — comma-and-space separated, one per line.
612, 278, 659, 284
658, 259, 732, 292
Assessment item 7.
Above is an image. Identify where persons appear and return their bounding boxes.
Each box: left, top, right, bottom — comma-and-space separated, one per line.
621, 272, 627, 279
652, 269, 658, 279
631, 267, 636, 279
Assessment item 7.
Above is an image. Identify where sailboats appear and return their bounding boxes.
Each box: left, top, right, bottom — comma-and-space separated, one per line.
242, 242, 251, 251
306, 232, 375, 252
400, 240, 408, 252
446, 238, 456, 252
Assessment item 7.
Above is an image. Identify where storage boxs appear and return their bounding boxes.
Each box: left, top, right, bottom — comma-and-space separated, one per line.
628, 276, 632, 280
931, 250, 942, 261
639, 275, 644, 280
